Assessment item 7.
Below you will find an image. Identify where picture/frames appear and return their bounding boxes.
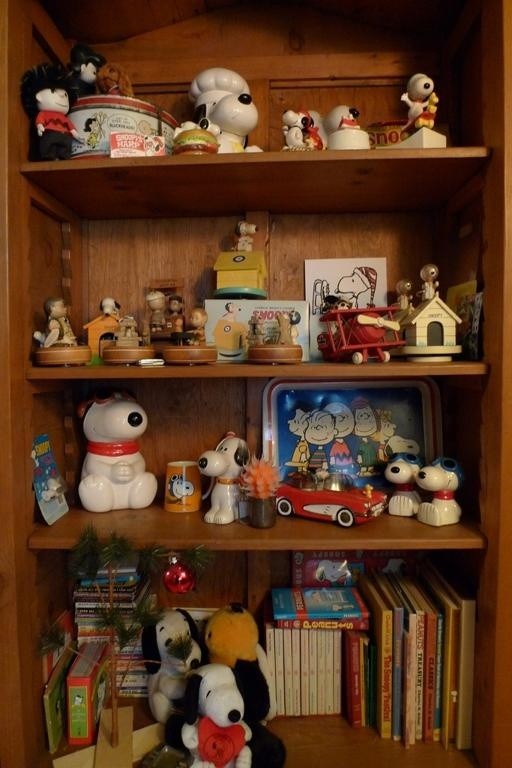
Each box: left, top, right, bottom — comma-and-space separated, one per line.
260, 378, 448, 492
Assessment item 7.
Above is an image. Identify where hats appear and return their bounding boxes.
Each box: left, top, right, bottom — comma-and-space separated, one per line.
353, 266, 378, 307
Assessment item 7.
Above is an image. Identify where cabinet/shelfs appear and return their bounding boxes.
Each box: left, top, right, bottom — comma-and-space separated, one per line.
1, 1, 509, 768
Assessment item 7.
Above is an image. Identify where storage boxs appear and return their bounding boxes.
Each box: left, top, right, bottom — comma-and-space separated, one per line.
198, 296, 314, 362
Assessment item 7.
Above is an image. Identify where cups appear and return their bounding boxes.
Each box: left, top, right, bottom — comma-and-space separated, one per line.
164, 460, 215, 516
